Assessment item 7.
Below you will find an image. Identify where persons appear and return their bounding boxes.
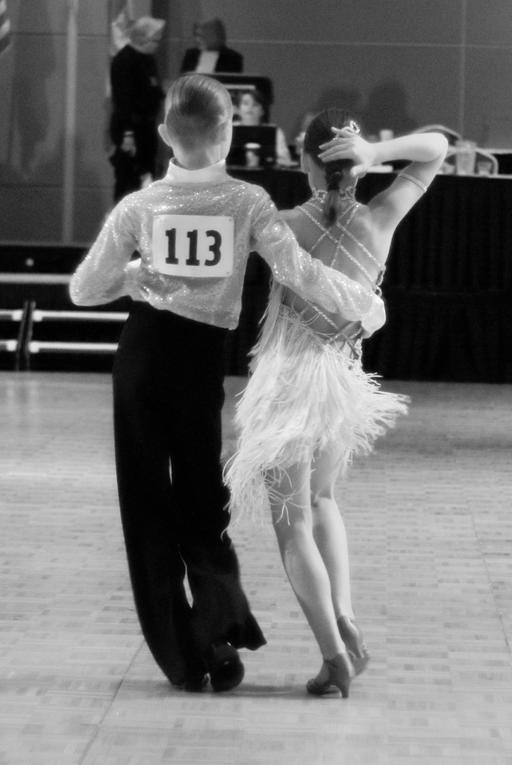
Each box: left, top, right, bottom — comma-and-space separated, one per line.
101, 12, 173, 208
176, 17, 246, 82
218, 86, 295, 174
219, 102, 451, 702
68, 72, 390, 698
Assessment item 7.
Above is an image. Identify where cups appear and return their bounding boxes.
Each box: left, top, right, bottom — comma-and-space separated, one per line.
478, 161, 492, 177
456, 140, 477, 176
380, 128, 393, 142
243, 143, 262, 167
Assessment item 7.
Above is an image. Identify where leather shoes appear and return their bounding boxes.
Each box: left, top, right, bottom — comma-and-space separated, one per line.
210, 645, 240, 690
228, 615, 265, 651
338, 615, 369, 676
306, 653, 354, 698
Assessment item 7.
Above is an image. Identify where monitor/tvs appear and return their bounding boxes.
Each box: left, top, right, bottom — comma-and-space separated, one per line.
226, 127, 276, 168
